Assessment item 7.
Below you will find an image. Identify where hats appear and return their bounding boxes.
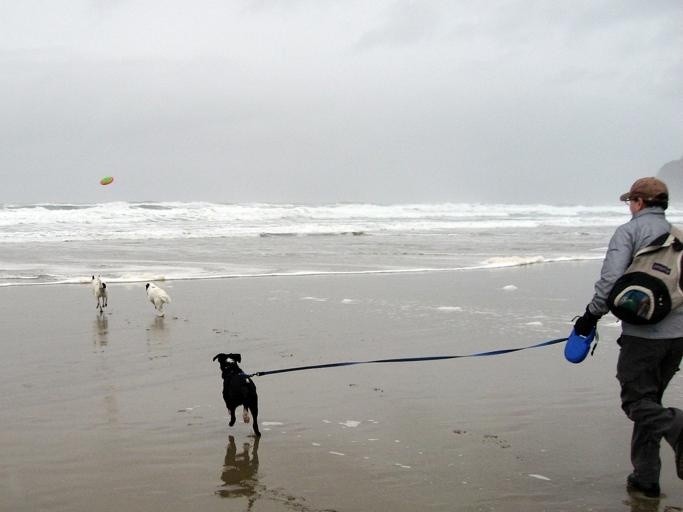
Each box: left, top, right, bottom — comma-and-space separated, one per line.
620, 177, 669, 204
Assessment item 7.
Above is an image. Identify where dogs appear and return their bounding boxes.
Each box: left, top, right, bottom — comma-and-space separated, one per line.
145, 281, 171, 318
91, 274, 108, 315
213, 352, 261, 436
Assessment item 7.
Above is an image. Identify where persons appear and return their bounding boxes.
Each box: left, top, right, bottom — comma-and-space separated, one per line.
573, 178, 683, 498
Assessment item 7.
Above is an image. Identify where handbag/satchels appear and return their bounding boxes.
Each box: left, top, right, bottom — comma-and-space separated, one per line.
606, 225, 683, 327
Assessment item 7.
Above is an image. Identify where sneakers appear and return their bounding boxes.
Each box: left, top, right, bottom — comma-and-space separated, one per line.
676, 431, 683, 481
626, 469, 660, 497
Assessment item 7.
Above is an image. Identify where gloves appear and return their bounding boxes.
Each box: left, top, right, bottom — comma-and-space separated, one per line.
575, 308, 597, 337
